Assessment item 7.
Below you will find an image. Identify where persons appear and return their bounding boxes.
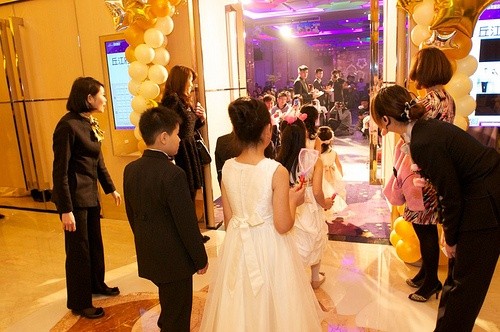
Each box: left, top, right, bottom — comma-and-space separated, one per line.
369, 84, 500, 332
275, 113, 327, 290
119, 106, 211, 332
218, 96, 307, 332
160, 65, 211, 245
214, 128, 278, 198
397, 48, 456, 303
243, 63, 371, 149
313, 125, 347, 216
52, 74, 123, 318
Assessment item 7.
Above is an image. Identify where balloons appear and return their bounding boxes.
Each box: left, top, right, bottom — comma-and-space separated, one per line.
387, 2, 478, 263
121, 1, 181, 156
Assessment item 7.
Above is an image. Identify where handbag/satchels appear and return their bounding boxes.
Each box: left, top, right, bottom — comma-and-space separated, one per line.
195, 128, 212, 165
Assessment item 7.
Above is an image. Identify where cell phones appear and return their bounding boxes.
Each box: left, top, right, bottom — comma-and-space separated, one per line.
196, 102, 201, 116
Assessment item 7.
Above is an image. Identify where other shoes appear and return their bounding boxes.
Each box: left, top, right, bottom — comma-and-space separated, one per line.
201, 234, 211, 243
72, 305, 104, 318
311, 271, 326, 289
91, 282, 120, 296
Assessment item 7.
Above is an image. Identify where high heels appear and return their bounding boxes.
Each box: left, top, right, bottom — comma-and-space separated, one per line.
408, 280, 442, 302
406, 277, 423, 287
